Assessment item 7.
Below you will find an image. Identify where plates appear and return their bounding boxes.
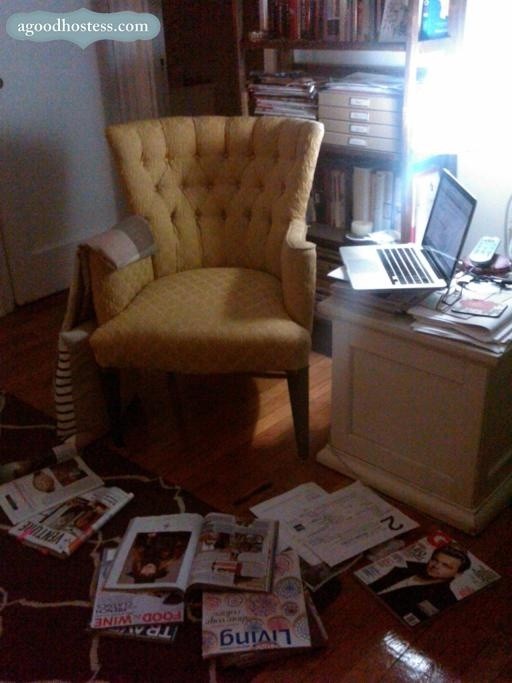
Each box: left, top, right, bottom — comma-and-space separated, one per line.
345, 233, 377, 241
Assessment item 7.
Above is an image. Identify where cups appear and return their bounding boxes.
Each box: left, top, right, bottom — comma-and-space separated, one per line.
351, 220, 372, 238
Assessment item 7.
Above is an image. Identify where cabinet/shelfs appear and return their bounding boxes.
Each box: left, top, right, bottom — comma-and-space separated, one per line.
231, 0, 463, 350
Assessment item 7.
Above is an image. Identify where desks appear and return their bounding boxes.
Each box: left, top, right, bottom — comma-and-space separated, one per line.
315, 297, 511, 541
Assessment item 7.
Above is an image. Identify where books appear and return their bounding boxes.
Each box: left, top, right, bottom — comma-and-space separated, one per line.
247, 0, 511, 355
0, 453, 502, 659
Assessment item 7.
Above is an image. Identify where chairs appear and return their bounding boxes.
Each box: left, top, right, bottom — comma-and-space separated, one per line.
80, 112, 322, 449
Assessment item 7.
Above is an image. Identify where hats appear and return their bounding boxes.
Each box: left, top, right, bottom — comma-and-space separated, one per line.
125, 566, 169, 583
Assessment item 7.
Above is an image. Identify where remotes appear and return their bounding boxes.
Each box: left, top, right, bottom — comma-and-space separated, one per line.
469, 235, 501, 266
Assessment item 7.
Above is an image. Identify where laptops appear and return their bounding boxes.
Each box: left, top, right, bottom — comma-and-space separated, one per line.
339, 167, 478, 292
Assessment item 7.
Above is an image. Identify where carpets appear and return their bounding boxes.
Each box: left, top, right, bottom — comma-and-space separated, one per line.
0, 387, 341, 683
